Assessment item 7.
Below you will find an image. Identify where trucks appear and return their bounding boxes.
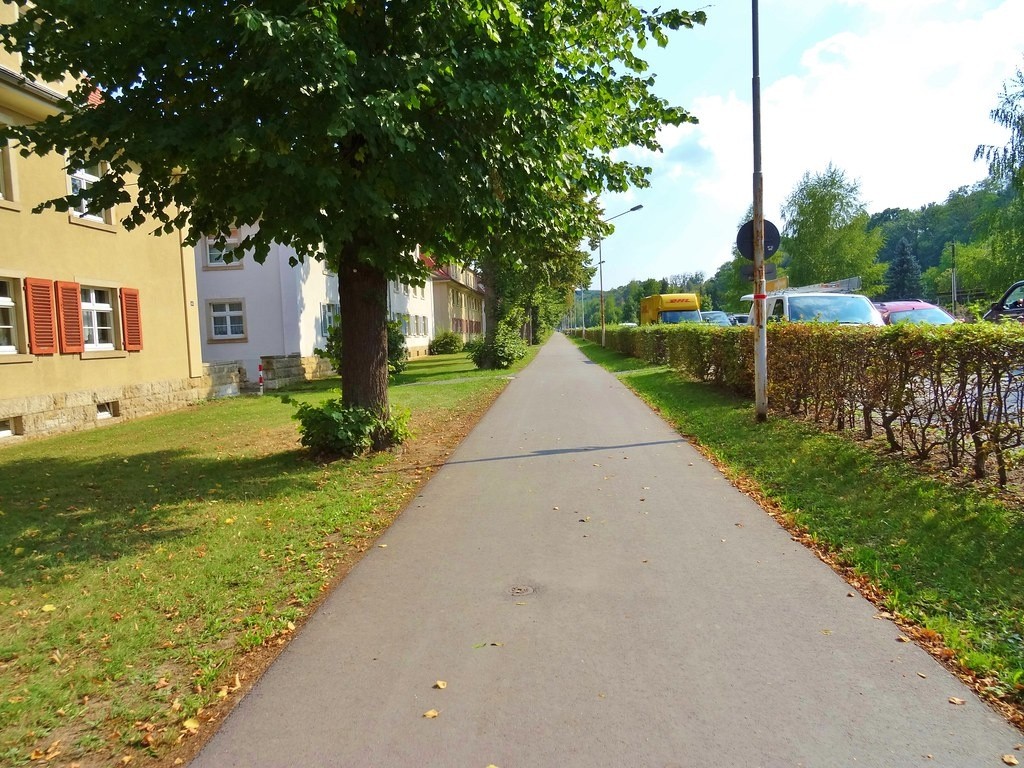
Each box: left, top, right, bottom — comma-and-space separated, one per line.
640, 293, 710, 330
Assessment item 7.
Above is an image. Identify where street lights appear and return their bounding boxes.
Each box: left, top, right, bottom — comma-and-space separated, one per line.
581, 261, 607, 342
597, 205, 644, 349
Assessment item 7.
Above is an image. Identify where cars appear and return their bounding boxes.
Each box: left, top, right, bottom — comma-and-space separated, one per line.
874, 299, 968, 359
980, 281, 1024, 327
701, 311, 751, 329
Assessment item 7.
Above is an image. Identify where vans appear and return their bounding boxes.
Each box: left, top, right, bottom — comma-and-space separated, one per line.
740, 293, 887, 342
